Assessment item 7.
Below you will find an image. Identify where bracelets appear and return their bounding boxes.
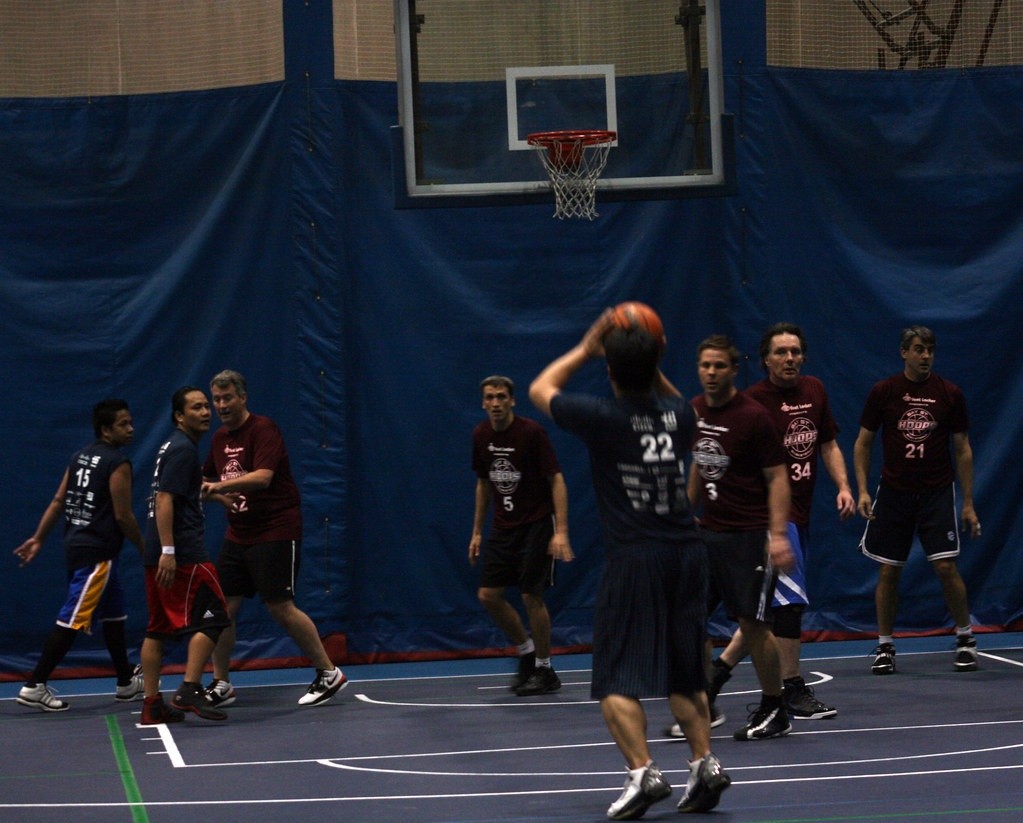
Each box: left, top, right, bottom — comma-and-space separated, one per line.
162, 546, 175, 555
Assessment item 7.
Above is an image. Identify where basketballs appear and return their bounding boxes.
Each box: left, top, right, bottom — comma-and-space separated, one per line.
602, 301, 664, 363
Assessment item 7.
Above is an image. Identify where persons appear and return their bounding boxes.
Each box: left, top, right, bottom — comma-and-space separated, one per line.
201, 370, 349, 707
468, 375, 573, 696
13, 399, 161, 711
853, 326, 981, 675
529, 308, 730, 820
706, 323, 856, 720
665, 335, 795, 739
141, 386, 232, 725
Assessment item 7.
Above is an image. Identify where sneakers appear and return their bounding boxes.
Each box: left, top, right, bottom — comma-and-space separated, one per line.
115, 675, 161, 702
139, 692, 186, 725
676, 751, 731, 813
509, 649, 537, 691
16, 683, 71, 712
867, 641, 896, 675
169, 685, 229, 721
515, 665, 561, 697
298, 665, 349, 706
203, 678, 236, 708
605, 759, 672, 820
732, 701, 793, 742
782, 678, 838, 721
948, 633, 979, 671
668, 704, 726, 737
